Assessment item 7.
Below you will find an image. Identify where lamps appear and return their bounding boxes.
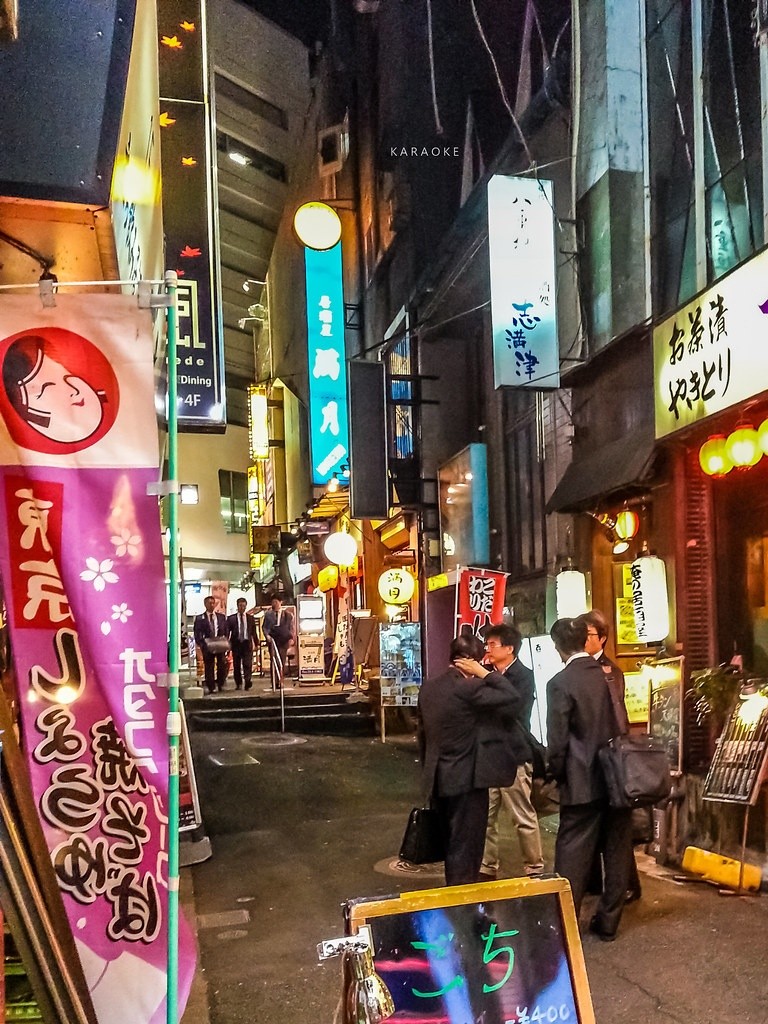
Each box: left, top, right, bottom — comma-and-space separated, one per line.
725, 410, 763, 470
698, 419, 733, 478
238, 318, 263, 331
179, 483, 199, 504
323, 522, 358, 565
289, 464, 347, 534
242, 277, 265, 292
378, 568, 415, 607
615, 507, 639, 541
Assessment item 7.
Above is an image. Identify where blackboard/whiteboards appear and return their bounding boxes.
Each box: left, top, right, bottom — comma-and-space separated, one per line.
342, 871, 596, 1024
649, 656, 684, 777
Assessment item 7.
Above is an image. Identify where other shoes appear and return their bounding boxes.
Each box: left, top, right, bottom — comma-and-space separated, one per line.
218, 684, 222, 691
237, 684, 242, 689
209, 689, 214, 694
623, 888, 640, 903
589, 914, 616, 942
245, 681, 252, 691
477, 872, 495, 882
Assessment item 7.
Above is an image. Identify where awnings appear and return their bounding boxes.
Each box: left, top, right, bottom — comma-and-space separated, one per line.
544, 427, 661, 517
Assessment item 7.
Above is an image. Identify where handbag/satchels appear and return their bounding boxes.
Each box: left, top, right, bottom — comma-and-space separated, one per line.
204, 635, 232, 654
399, 799, 446, 865
598, 734, 672, 808
530, 776, 561, 818
532, 739, 554, 779
269, 626, 293, 647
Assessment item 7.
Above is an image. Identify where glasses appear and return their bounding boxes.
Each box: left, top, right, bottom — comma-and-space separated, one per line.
484, 645, 506, 651
587, 633, 599, 642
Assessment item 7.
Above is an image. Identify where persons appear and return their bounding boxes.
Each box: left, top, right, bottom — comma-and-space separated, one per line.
417, 633, 523, 885
262, 595, 293, 690
226, 598, 261, 691
475, 624, 545, 880
578, 610, 641, 904
194, 596, 227, 693
547, 618, 631, 942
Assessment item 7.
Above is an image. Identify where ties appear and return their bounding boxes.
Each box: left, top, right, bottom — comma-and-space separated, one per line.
240, 614, 244, 642
210, 614, 215, 638
276, 611, 279, 626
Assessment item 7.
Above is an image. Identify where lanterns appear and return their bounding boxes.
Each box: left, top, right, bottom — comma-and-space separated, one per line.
556, 566, 586, 620
378, 568, 415, 603
615, 508, 639, 541
725, 425, 764, 471
631, 549, 670, 646
698, 434, 734, 478
318, 532, 358, 593
759, 420, 768, 454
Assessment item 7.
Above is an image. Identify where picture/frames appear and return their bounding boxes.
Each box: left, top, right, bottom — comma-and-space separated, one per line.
623, 672, 648, 723
